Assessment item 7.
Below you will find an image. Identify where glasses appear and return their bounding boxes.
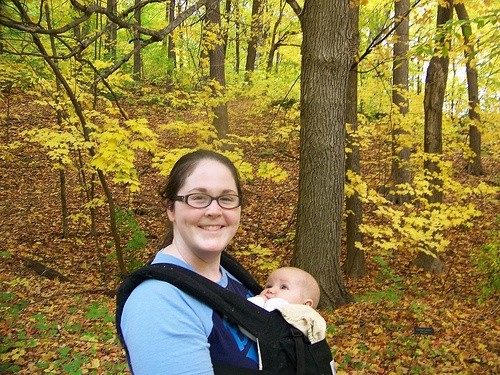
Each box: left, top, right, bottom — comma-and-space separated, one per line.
171, 193, 243, 209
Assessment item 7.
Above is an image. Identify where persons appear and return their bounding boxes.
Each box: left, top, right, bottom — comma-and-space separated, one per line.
115, 148, 262, 375
237, 267, 337, 375
